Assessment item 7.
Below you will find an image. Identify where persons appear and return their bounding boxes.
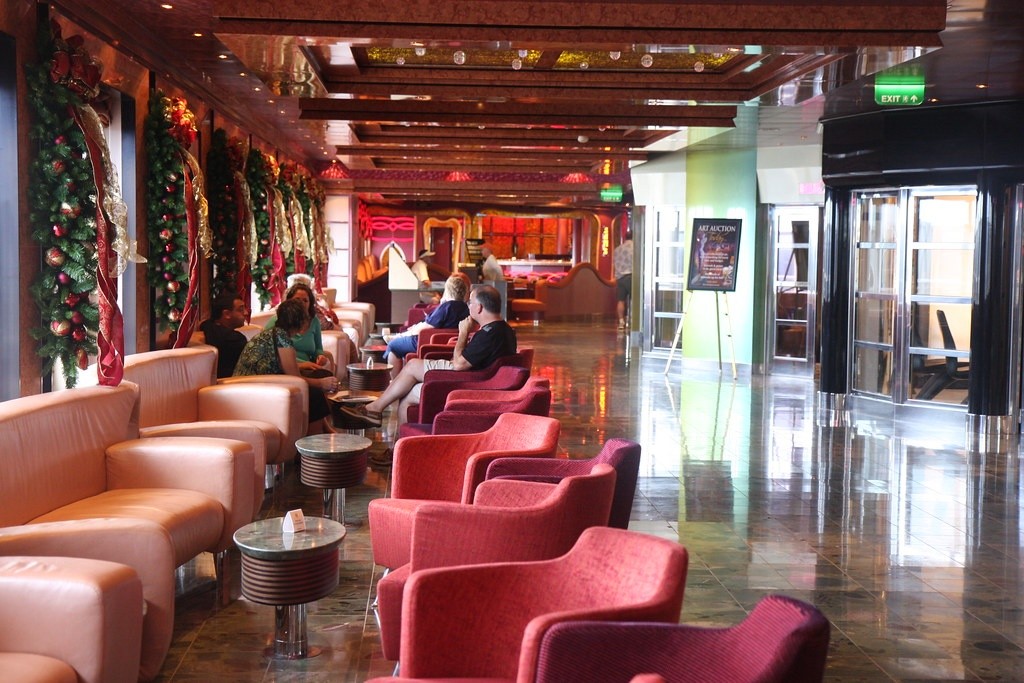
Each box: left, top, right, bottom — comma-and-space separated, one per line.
614, 229, 633, 329
340, 284, 518, 466
199, 283, 341, 435
382, 244, 503, 381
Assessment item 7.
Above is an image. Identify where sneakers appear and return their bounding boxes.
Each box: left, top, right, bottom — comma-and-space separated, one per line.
340, 404, 383, 428
370, 448, 393, 465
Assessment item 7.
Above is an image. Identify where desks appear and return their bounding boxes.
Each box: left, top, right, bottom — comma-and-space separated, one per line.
325, 389, 384, 438
375, 323, 404, 335
345, 363, 394, 391
359, 346, 388, 365
231, 515, 347, 661
294, 434, 372, 529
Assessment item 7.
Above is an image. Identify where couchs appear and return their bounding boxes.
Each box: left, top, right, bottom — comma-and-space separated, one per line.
515, 263, 617, 322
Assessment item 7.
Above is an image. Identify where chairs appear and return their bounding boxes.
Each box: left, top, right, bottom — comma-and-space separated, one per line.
910, 310, 970, 402
356, 254, 388, 284
0, 301, 831, 683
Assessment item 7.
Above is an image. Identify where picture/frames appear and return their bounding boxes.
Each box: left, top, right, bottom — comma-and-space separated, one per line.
687, 216, 742, 291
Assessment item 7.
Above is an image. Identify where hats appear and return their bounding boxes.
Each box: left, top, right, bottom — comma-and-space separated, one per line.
418, 250, 435, 259
480, 243, 493, 252
